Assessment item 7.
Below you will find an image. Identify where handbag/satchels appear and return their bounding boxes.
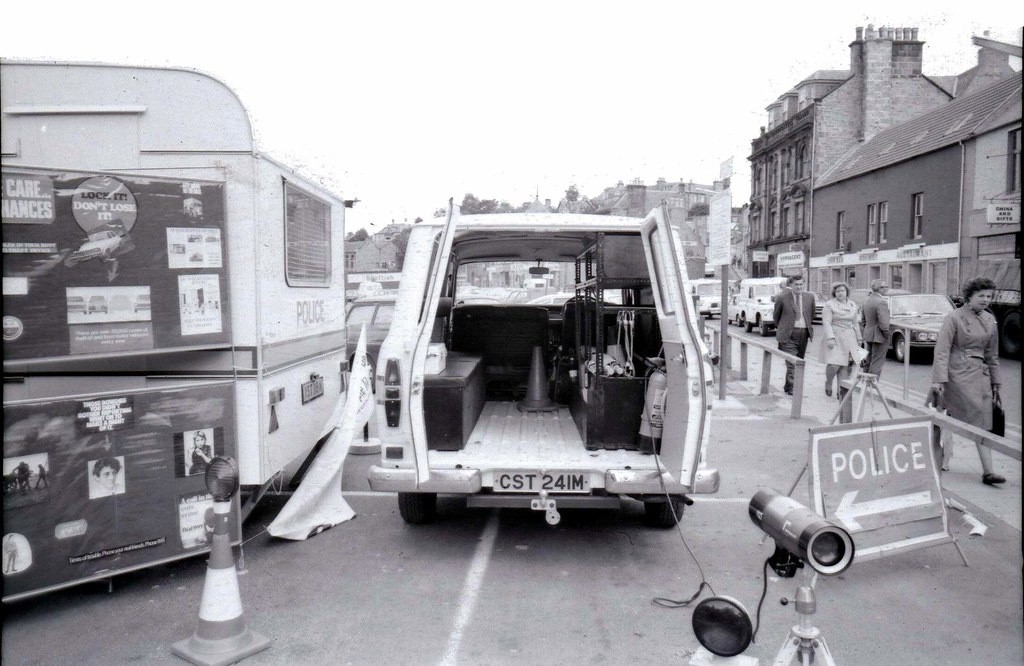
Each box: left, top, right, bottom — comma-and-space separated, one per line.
988, 390, 1006, 437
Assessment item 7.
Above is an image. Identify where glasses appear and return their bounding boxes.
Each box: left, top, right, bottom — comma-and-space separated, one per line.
880, 285, 888, 289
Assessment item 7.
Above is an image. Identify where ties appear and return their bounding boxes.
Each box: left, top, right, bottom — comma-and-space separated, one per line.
795, 294, 802, 321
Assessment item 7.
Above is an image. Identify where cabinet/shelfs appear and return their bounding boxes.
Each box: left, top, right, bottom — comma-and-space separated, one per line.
565, 233, 667, 452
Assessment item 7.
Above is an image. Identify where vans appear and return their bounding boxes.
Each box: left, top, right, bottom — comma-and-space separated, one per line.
364, 196, 721, 530
738, 278, 789, 336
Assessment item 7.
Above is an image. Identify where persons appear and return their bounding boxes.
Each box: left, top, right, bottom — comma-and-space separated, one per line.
820, 282, 862, 400
932, 277, 1007, 484
861, 280, 892, 386
773, 275, 816, 395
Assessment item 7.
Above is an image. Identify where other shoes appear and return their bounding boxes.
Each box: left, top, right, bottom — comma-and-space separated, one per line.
784, 385, 794, 395
824, 381, 833, 396
982, 473, 1006, 484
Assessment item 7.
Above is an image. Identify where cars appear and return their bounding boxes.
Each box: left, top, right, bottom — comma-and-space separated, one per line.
848, 289, 911, 306
807, 291, 830, 324
727, 294, 742, 328
692, 278, 732, 319
858, 293, 959, 364
454, 285, 624, 316
343, 298, 398, 398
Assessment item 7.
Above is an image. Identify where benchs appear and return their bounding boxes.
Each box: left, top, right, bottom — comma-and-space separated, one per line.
422, 351, 486, 451
453, 304, 553, 398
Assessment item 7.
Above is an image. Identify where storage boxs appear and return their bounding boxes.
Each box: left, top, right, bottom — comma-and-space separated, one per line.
425, 343, 448, 375
